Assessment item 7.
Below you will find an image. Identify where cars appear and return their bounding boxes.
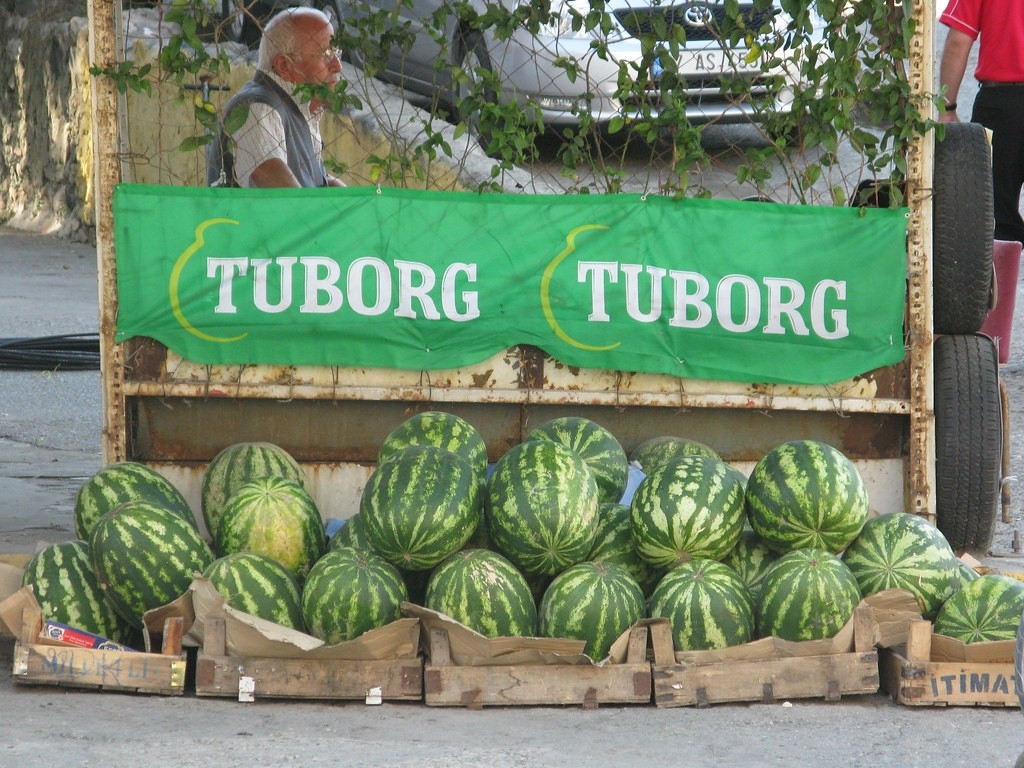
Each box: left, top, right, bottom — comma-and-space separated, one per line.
219, 1, 837, 160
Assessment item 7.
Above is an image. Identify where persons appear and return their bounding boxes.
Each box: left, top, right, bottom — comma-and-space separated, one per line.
937, 0, 1024, 253
206, 6, 351, 188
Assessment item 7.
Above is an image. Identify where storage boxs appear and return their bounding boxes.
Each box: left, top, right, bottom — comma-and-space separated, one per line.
12, 607, 1021, 708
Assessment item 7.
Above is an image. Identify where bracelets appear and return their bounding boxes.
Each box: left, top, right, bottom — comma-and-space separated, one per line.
945, 103, 957, 111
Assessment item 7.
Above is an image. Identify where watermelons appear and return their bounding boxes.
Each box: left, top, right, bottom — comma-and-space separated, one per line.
19, 412, 1024, 666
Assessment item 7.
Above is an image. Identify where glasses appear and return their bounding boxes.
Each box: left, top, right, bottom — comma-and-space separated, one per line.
285, 47, 343, 64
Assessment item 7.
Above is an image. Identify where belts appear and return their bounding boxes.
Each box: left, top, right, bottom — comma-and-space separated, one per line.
982, 81, 1024, 89
